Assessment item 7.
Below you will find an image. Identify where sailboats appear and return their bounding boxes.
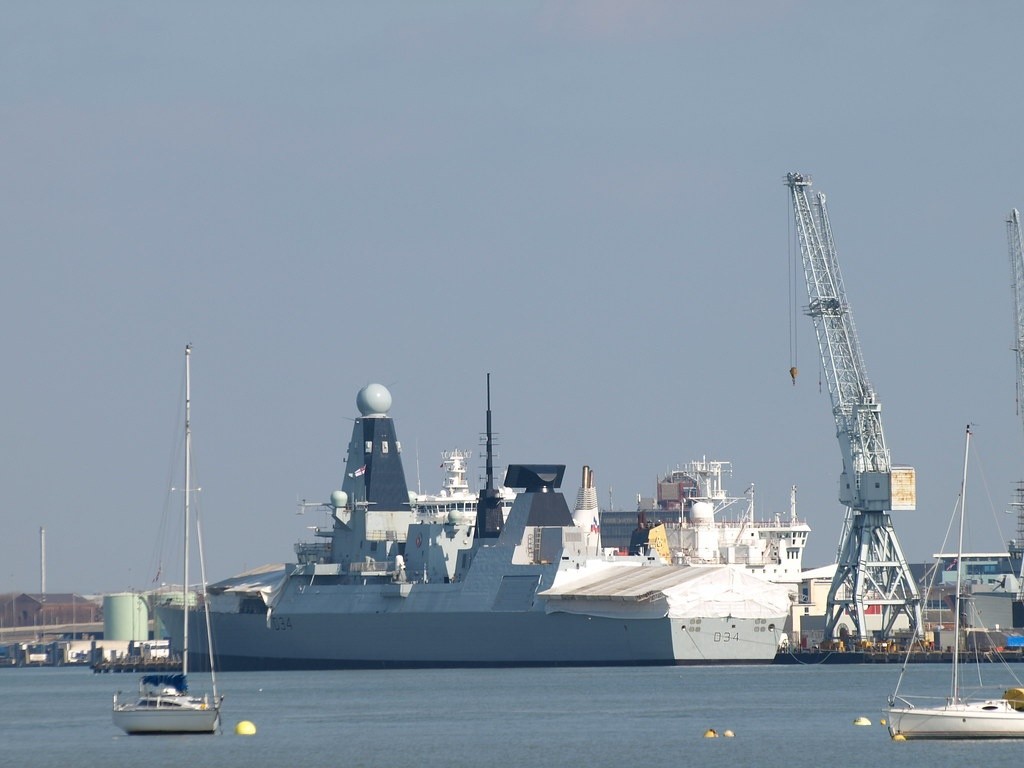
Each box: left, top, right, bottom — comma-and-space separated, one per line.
110, 343, 225, 737
877, 423, 1024, 738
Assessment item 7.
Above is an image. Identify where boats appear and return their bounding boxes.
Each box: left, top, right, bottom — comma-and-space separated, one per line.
151, 373, 793, 664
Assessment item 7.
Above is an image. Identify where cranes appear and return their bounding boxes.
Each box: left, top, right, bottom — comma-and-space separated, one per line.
1002, 206, 1024, 603
777, 171, 929, 653
